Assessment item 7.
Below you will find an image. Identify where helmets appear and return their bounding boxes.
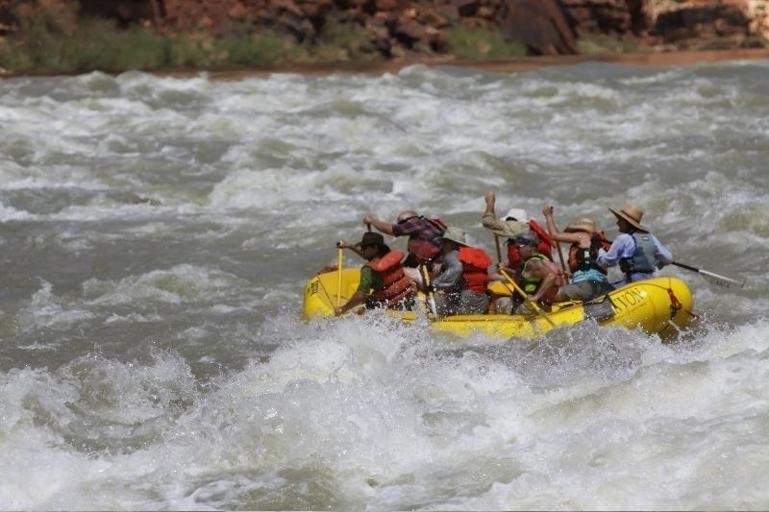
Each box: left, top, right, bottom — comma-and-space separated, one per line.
396, 211, 420, 224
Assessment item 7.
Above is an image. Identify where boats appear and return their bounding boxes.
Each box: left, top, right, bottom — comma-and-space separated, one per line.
302, 267, 694, 340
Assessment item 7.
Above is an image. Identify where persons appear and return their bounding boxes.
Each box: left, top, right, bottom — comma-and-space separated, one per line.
409, 233, 491, 315
481, 189, 554, 282
333, 230, 417, 315
539, 203, 611, 302
577, 204, 674, 296
361, 209, 447, 289
493, 231, 557, 315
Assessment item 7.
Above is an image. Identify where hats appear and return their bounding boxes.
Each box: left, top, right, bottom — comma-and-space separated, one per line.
498, 208, 530, 224
433, 228, 476, 249
354, 231, 384, 247
608, 203, 651, 235
563, 217, 597, 234
